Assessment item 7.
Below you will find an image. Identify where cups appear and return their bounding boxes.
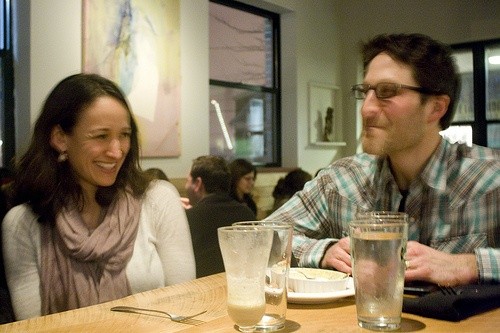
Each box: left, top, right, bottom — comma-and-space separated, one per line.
349, 211, 409, 330
218, 226, 275, 333
231, 221, 293, 332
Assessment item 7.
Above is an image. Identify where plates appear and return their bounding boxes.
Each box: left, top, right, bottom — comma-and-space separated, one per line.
289, 267, 356, 305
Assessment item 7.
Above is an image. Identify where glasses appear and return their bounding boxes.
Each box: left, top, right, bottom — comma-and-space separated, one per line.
352, 82, 437, 100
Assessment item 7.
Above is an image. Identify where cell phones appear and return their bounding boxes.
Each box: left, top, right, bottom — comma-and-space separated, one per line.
404, 280, 437, 295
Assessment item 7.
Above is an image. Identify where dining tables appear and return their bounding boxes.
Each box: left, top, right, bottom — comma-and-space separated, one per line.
0, 271, 500, 333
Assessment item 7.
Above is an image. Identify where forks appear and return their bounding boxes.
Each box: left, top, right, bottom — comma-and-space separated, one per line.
110, 306, 208, 322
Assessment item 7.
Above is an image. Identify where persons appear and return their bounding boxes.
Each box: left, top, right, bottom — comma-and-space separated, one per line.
252, 32, 500, 289
181, 157, 253, 279
0, 74, 197, 322
229, 160, 258, 220
272, 169, 313, 213
